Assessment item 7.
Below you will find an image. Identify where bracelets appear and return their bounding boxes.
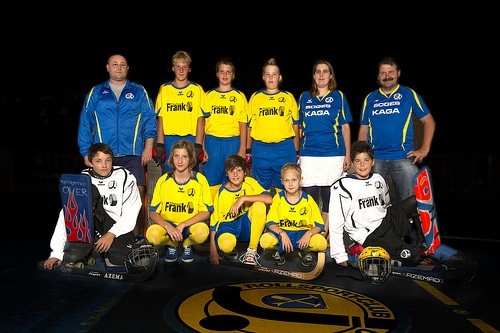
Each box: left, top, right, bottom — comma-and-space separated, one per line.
278, 229, 284, 235
295, 150, 300, 155
245, 148, 252, 154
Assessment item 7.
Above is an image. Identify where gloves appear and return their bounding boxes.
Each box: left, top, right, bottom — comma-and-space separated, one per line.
156, 143, 166, 164
194, 143, 204, 162
245, 154, 252, 169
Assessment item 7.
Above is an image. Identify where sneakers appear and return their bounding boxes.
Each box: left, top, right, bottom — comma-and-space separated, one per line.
239, 251, 261, 267
224, 249, 238, 260
298, 251, 316, 266
181, 246, 194, 263
165, 246, 179, 262
272, 250, 286, 265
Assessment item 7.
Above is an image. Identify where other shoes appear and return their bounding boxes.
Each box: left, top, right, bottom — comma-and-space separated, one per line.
126, 261, 146, 273
391, 260, 402, 267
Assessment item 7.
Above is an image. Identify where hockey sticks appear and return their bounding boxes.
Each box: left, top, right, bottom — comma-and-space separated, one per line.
346, 259, 449, 286
37, 249, 159, 284
206, 251, 326, 282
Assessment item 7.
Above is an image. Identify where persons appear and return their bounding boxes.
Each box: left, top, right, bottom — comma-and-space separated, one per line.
244, 52, 299, 252
192, 56, 248, 249
78, 52, 158, 244
260, 162, 328, 267
357, 56, 436, 256
145, 140, 214, 263
44, 142, 142, 276
328, 140, 427, 267
296, 54, 354, 247
154, 50, 208, 250
209, 155, 272, 266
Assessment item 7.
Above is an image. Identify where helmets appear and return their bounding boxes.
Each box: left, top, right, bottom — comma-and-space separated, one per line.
358, 246, 391, 282
